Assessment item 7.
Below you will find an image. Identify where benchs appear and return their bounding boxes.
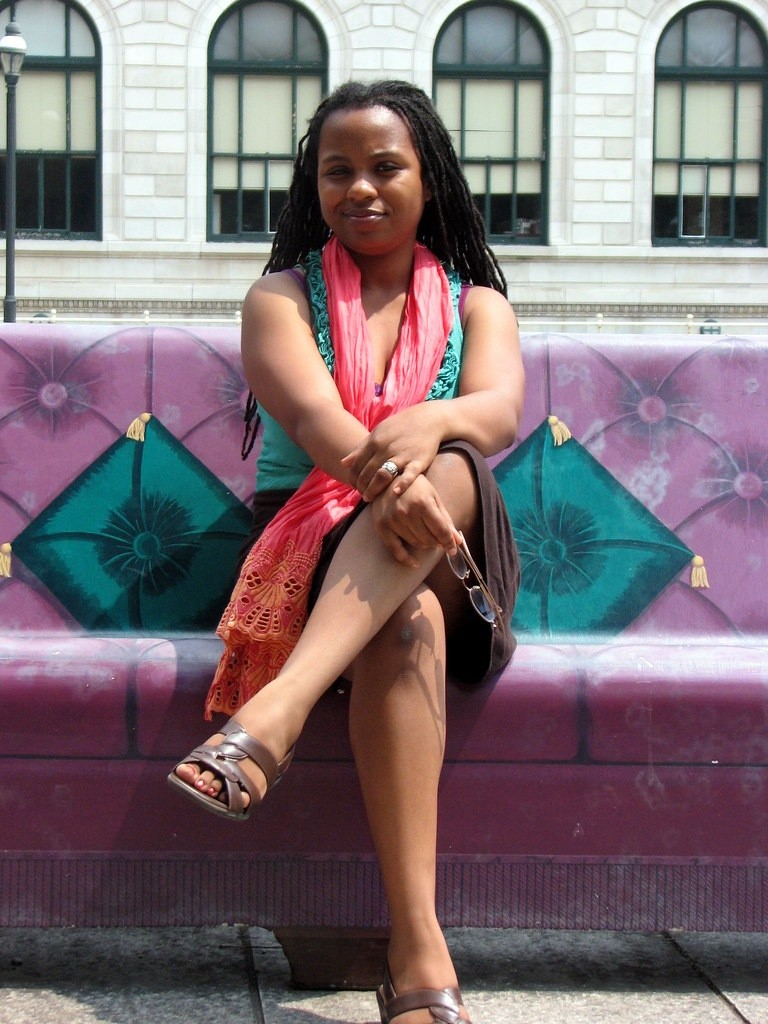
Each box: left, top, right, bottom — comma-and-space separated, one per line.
0, 320, 768, 987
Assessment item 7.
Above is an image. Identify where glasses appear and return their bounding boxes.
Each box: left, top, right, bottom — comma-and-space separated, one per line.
446, 530, 504, 628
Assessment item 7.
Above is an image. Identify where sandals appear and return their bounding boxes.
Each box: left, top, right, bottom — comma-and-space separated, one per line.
376, 953, 472, 1024
167, 718, 296, 821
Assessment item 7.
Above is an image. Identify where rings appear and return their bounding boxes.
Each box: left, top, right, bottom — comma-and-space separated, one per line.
381, 461, 398, 478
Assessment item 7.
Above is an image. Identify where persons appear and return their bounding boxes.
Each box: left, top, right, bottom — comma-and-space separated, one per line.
167, 80, 526, 1024
